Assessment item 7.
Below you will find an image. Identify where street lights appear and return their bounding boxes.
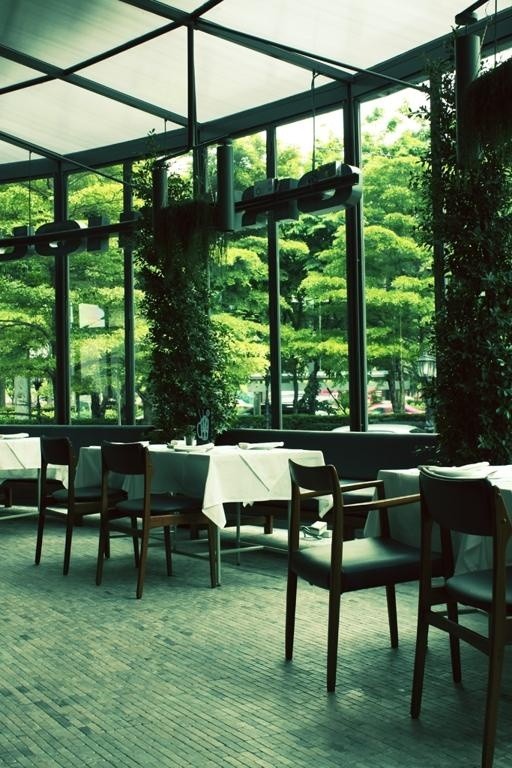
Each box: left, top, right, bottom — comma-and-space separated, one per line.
414, 350, 437, 432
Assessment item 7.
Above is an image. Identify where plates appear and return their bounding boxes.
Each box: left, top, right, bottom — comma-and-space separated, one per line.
429, 465, 498, 480
239, 441, 281, 450
175, 446, 213, 454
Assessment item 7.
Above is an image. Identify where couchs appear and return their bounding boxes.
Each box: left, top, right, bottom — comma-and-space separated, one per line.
0, 423, 157, 506
209, 427, 443, 541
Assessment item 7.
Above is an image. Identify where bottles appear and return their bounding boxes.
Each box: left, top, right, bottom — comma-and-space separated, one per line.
195, 407, 212, 445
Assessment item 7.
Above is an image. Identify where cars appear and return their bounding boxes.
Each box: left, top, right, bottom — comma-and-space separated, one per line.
234, 387, 421, 417
328, 422, 429, 434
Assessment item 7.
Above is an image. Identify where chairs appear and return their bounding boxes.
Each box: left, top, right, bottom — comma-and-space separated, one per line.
93, 440, 216, 599
408, 466, 511, 766
280, 458, 465, 693
34, 433, 140, 577
